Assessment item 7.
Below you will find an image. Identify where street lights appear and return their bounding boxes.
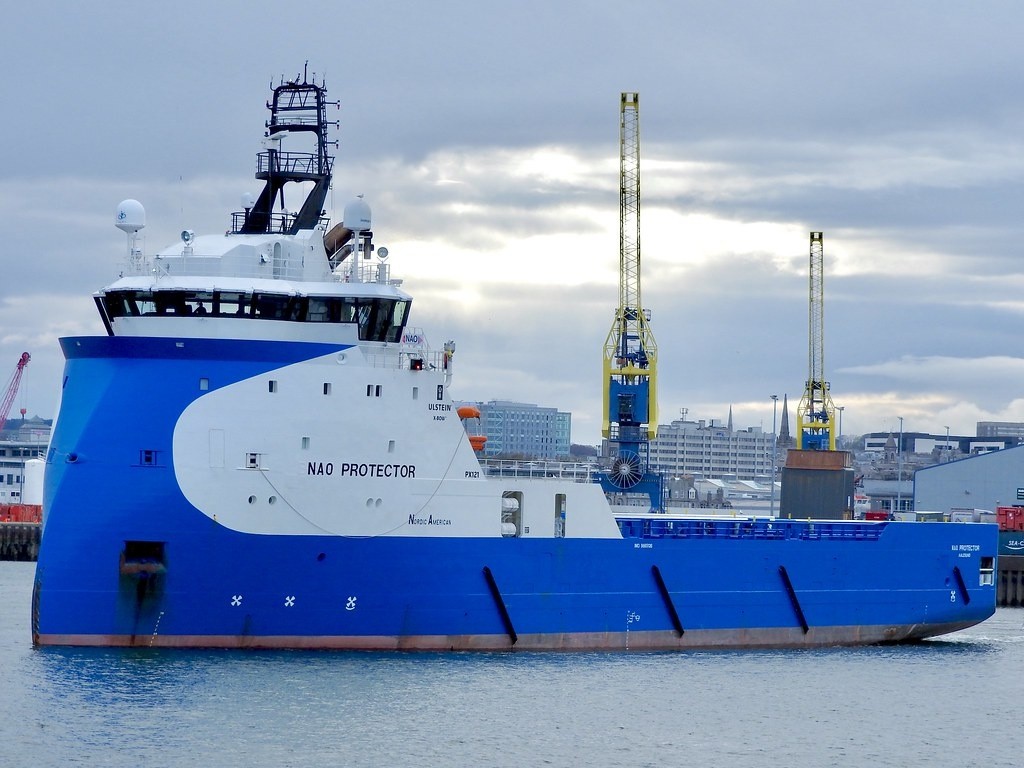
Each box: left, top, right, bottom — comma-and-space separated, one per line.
768, 394, 778, 517
836, 406, 845, 436
897, 416, 905, 511
945, 426, 950, 463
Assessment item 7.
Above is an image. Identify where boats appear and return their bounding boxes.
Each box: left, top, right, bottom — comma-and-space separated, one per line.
29, 56, 1001, 652
